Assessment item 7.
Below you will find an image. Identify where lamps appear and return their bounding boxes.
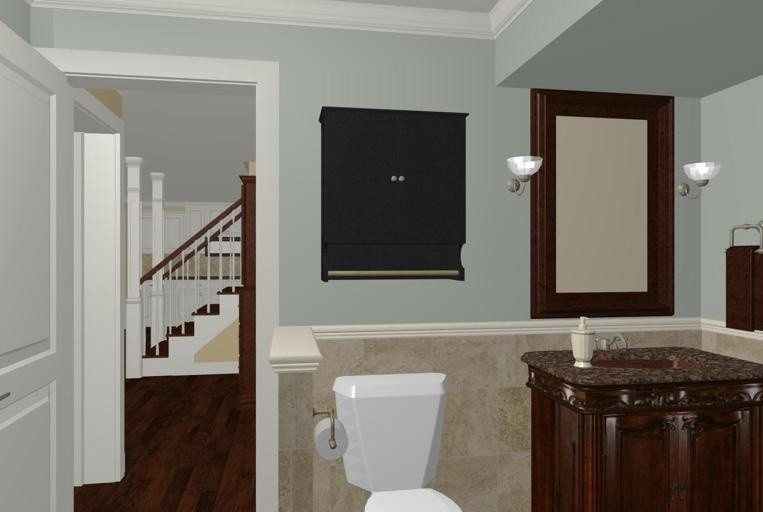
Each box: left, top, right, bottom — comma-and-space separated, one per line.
505, 156, 544, 196
678, 161, 720, 199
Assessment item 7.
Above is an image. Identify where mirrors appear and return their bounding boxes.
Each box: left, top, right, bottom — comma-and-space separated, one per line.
529, 87, 675, 317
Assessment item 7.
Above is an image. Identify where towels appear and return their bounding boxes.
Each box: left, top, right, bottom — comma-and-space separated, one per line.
725, 245, 763, 332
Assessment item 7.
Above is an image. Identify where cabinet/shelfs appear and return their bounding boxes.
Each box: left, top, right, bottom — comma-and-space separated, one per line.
318, 105, 470, 283
